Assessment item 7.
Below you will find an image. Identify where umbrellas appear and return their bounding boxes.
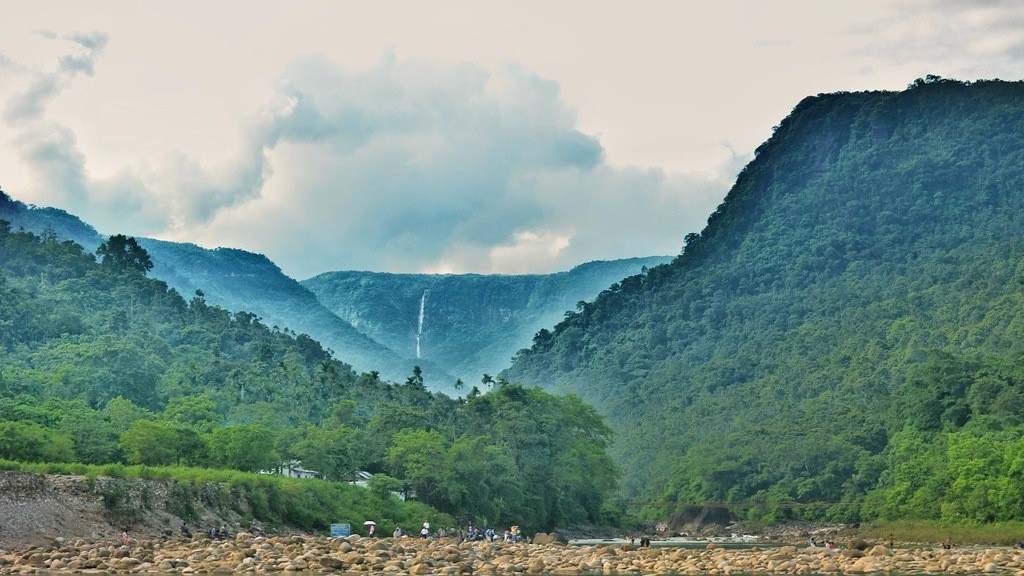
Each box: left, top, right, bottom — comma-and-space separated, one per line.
364, 521, 376, 525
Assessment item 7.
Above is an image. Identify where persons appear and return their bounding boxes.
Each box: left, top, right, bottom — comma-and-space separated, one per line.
627, 535, 635, 544
825, 541, 834, 548
505, 525, 533, 544
942, 538, 957, 549
421, 520, 430, 539
438, 525, 495, 542
182, 523, 189, 533
392, 528, 397, 538
1018, 541, 1024, 548
889, 534, 894, 546
810, 538, 817, 548
123, 529, 129, 539
209, 524, 227, 539
370, 524, 375, 538
640, 537, 650, 547
